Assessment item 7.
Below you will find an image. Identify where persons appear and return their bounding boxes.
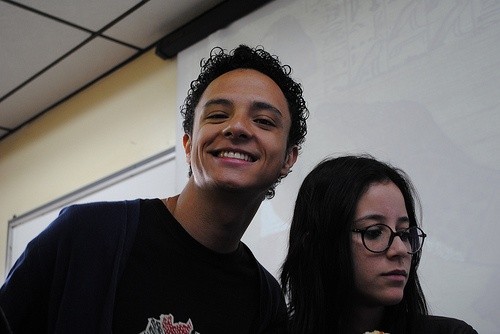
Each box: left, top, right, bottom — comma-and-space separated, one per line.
280, 151, 479, 334
0, 43, 311, 334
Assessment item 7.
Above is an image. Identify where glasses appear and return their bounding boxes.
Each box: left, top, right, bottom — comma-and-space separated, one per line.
352, 223, 426, 254
